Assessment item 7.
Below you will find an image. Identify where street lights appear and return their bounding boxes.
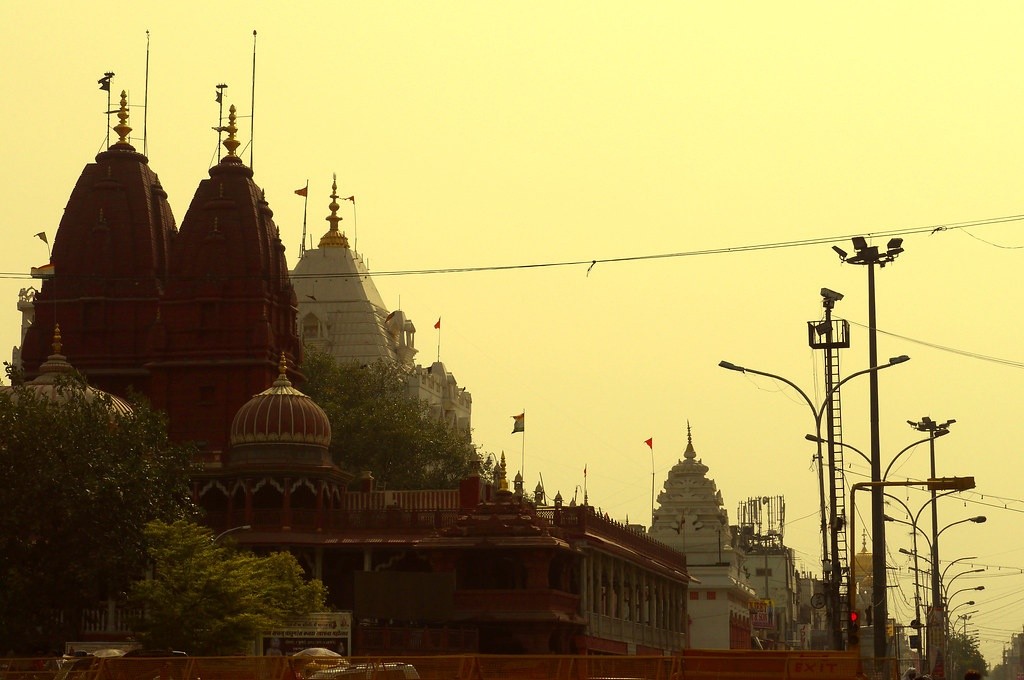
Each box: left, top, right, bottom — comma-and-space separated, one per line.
718, 231, 989, 677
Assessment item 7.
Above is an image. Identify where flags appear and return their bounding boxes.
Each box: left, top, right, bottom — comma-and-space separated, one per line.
645, 438, 652, 450
435, 320, 440, 329
510, 412, 524, 433
344, 195, 354, 204
295, 186, 307, 198
33, 231, 48, 244
